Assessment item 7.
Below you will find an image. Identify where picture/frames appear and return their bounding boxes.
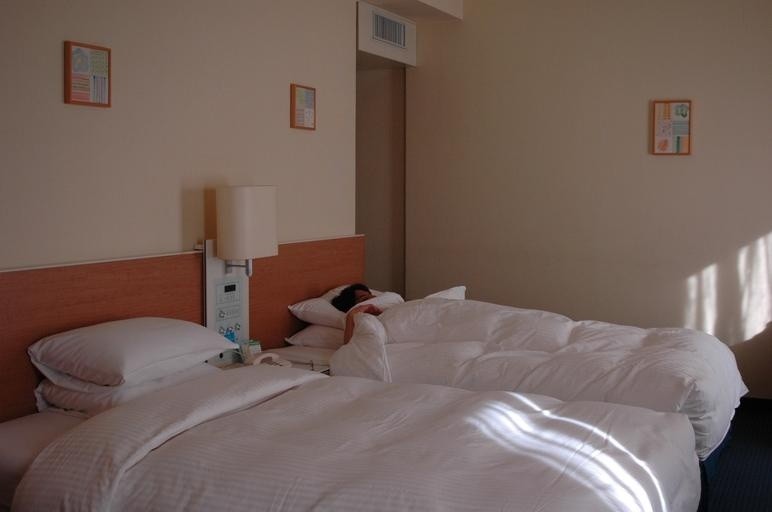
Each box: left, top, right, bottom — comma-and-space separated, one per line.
289, 82, 317, 132
651, 99, 692, 157
63, 39, 113, 110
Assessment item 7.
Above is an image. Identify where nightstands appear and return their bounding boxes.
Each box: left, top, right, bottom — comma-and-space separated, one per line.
215, 360, 334, 374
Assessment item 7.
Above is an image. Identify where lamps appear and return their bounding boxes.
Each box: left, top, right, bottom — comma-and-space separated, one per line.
213, 183, 281, 277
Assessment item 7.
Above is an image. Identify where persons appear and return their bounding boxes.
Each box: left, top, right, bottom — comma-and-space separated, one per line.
332, 281, 387, 344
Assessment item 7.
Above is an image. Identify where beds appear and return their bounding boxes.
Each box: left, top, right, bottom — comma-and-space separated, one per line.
262, 292, 751, 465
0, 361, 702, 511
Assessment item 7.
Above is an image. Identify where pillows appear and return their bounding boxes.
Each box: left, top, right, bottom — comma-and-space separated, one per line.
29, 361, 224, 416
23, 314, 241, 394
287, 283, 387, 328
285, 323, 346, 350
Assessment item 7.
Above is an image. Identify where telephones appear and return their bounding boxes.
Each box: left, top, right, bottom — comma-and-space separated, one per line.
250, 352, 292, 369
